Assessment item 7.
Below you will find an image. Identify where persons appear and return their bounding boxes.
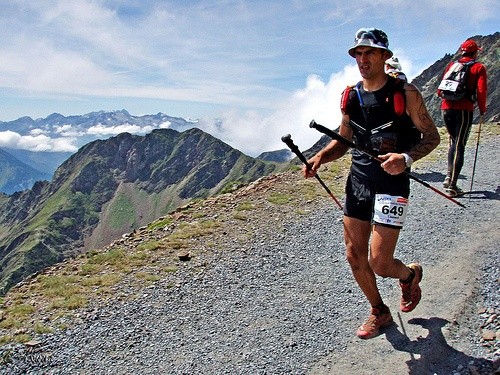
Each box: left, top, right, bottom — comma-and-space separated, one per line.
385, 57, 407, 82
303, 27, 441, 339
437, 40, 488, 197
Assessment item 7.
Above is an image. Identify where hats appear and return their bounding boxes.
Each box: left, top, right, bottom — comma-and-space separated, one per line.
385, 57, 402, 70
347, 26, 393, 59
461, 40, 482, 52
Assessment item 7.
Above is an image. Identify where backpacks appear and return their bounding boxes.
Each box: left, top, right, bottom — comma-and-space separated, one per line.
438, 58, 481, 100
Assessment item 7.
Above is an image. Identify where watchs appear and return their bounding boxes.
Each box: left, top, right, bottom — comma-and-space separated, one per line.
401, 153, 413, 168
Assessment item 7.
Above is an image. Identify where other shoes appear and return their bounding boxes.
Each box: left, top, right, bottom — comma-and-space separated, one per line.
442, 176, 464, 199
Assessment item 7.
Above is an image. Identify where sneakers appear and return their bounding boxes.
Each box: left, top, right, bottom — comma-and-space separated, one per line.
357, 304, 393, 339
398, 263, 423, 313
